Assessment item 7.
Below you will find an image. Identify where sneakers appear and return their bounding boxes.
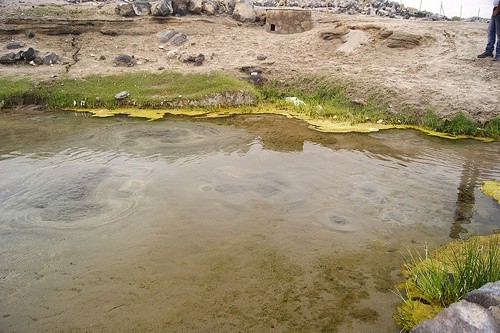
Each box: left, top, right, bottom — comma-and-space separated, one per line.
477, 50, 493, 58
492, 56, 500, 61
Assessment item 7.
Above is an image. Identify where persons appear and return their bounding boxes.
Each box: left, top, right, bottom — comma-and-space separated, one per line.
477, 0, 500, 61
